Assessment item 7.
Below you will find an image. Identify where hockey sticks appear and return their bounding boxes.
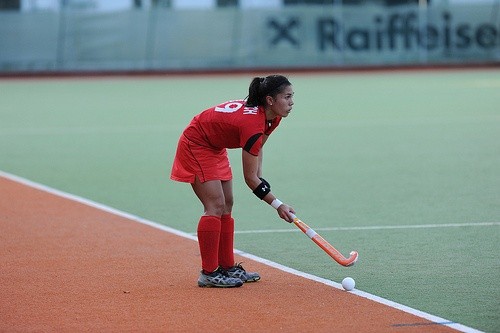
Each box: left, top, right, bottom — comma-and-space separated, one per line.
290, 210, 359, 268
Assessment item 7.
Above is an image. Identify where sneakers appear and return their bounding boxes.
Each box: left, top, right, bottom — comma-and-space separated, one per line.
227, 263, 260, 282
197, 268, 241, 287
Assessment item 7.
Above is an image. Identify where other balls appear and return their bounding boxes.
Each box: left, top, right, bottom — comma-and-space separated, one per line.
340, 276, 357, 291
